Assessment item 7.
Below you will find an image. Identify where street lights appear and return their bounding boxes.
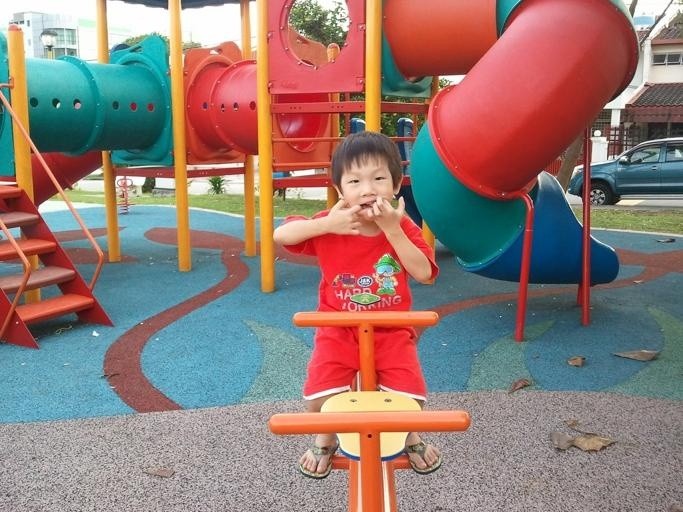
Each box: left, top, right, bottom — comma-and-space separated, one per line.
40, 29, 56, 61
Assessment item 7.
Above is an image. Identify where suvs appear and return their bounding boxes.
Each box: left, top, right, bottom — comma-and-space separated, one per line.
567, 136, 683, 207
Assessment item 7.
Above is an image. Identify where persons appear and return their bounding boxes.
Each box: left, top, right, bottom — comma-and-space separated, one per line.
272, 131, 443, 478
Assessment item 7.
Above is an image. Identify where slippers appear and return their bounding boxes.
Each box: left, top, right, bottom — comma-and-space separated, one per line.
404, 434, 442, 475
300, 441, 339, 479
